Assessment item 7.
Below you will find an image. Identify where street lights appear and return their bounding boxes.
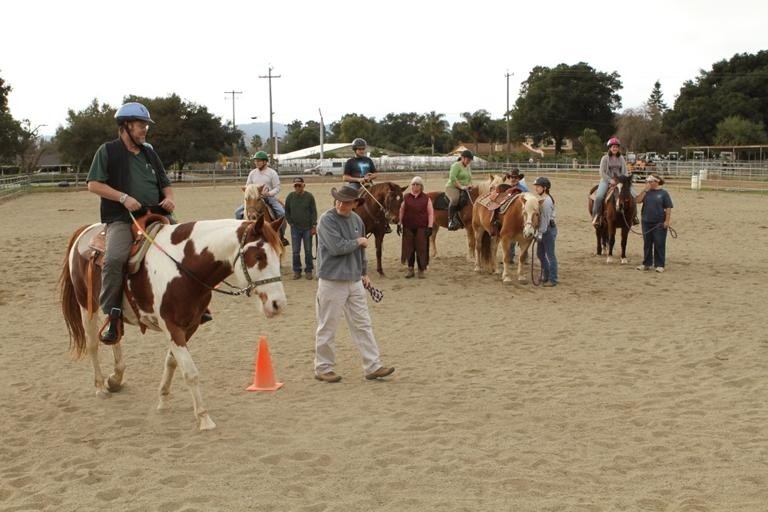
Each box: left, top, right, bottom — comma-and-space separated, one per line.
246, 335, 284, 391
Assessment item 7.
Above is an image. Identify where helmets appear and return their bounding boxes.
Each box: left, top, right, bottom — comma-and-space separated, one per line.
252, 151, 269, 161
533, 177, 551, 189
114, 102, 156, 126
351, 138, 366, 151
607, 138, 621, 148
461, 150, 473, 160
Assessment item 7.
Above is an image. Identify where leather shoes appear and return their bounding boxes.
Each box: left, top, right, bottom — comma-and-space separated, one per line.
103, 318, 124, 340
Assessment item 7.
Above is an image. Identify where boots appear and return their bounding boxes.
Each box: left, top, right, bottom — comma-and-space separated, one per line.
448, 205, 457, 228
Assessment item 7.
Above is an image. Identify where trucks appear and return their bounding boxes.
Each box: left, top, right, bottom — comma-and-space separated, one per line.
319, 161, 347, 176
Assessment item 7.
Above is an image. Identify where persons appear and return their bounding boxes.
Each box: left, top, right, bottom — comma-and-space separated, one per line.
285, 177, 317, 279
86, 102, 210, 345
499, 169, 531, 265
445, 150, 473, 231
235, 152, 290, 246
532, 177, 557, 287
397, 177, 434, 278
342, 138, 377, 189
592, 137, 640, 226
314, 186, 394, 382
634, 173, 673, 273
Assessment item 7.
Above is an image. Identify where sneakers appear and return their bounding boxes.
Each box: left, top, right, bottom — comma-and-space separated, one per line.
366, 367, 394, 380
306, 271, 313, 280
294, 272, 301, 280
418, 269, 425, 278
315, 372, 341, 382
404, 270, 414, 277
636, 264, 652, 270
656, 266, 665, 272
543, 280, 553, 287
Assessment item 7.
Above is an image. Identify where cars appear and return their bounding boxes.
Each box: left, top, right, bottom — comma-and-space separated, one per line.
304, 165, 321, 175
32, 166, 73, 173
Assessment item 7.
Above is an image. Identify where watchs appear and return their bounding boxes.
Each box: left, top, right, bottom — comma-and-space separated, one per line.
119, 193, 128, 204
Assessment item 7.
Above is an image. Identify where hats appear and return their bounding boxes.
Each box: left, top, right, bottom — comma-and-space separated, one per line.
293, 177, 305, 186
332, 187, 365, 208
504, 168, 524, 180
645, 175, 660, 183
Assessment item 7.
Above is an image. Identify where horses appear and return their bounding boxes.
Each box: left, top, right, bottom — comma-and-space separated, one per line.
425, 173, 507, 262
241, 182, 286, 237
333, 180, 409, 279
55, 213, 288, 439
588, 172, 635, 265
471, 191, 546, 286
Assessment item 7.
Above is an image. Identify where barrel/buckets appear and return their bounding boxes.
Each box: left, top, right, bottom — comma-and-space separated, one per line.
691, 176, 701, 190
699, 170, 707, 179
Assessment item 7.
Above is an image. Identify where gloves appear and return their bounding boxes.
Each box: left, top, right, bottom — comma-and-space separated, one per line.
534, 232, 543, 242
397, 224, 402, 235
425, 228, 432, 238
